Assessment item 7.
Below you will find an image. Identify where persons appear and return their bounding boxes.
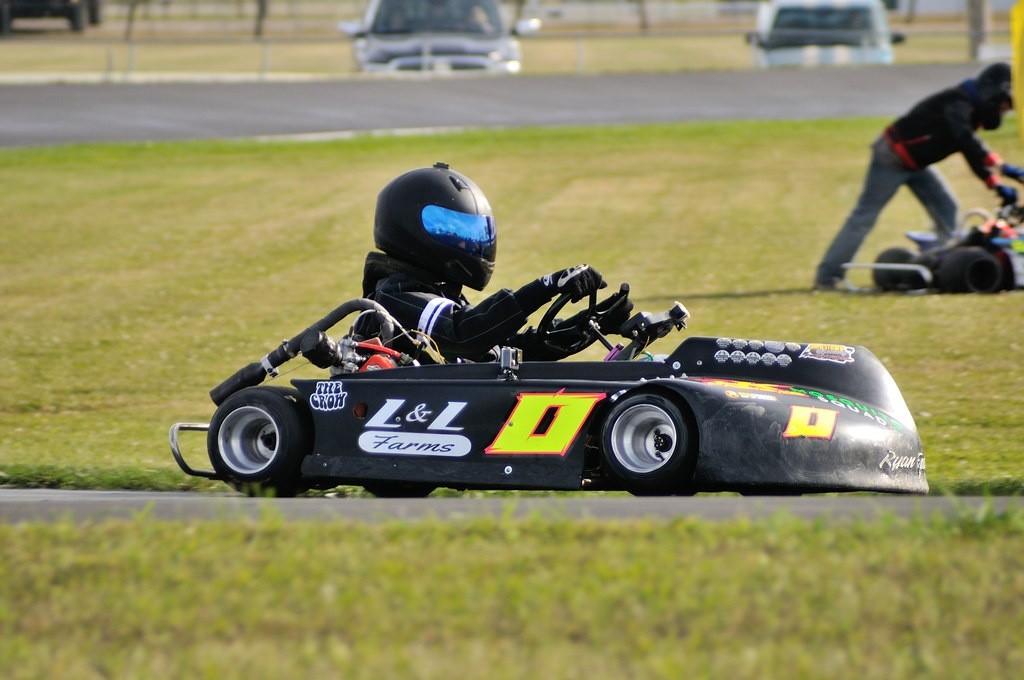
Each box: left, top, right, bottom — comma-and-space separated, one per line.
813, 62, 1024, 291
362, 163, 634, 365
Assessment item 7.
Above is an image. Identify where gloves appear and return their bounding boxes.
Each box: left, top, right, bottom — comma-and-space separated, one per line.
1002, 164, 1024, 183
586, 293, 634, 335
997, 185, 1016, 206
541, 263, 607, 303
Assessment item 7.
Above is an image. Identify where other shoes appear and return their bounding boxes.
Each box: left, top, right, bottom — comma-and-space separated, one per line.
811, 278, 873, 293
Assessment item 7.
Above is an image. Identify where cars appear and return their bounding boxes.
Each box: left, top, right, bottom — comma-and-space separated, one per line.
745, 1, 906, 70
0, 0, 103, 33
337, 1, 541, 75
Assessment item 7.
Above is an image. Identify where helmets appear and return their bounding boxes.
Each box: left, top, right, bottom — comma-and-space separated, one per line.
978, 63, 1012, 130
374, 161, 497, 291
